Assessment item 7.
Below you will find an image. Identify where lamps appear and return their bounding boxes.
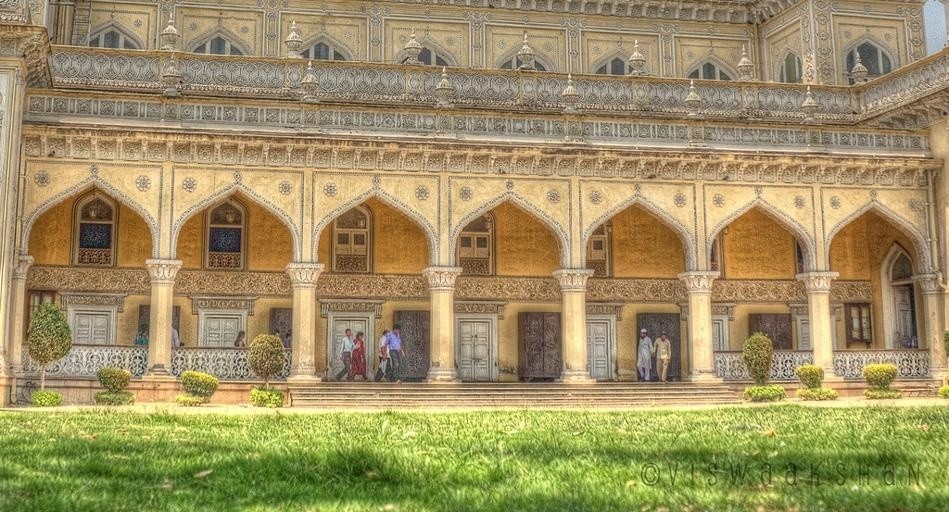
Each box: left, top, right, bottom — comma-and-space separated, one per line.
356, 203, 367, 230
89, 192, 99, 219
226, 196, 237, 223
484, 210, 492, 230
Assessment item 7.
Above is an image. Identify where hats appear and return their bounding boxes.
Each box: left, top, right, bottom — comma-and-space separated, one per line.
640, 328, 647, 333
